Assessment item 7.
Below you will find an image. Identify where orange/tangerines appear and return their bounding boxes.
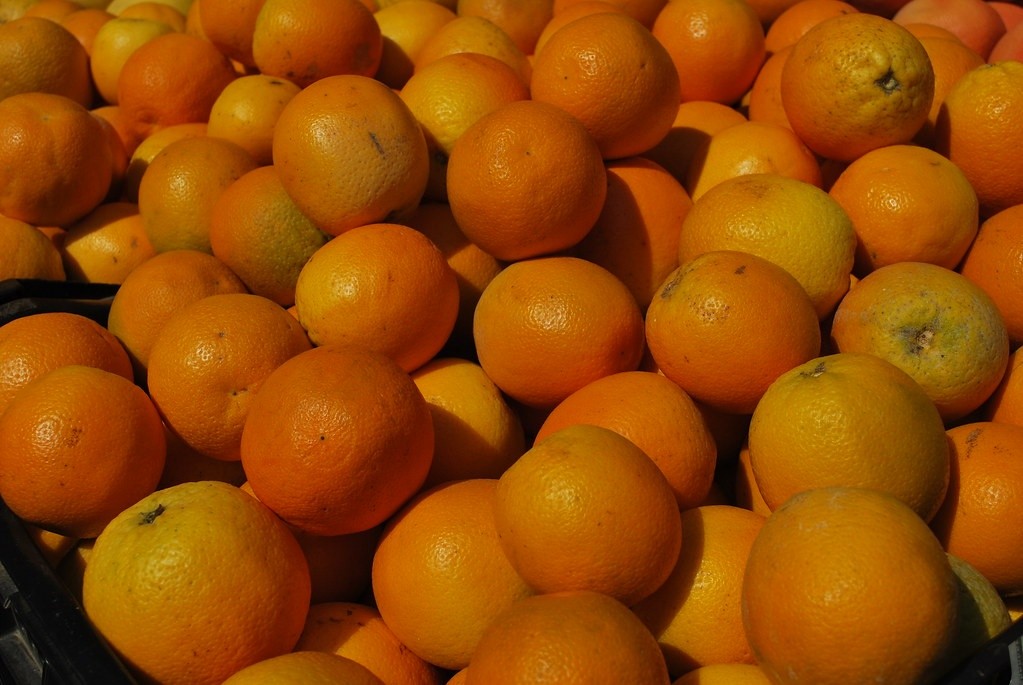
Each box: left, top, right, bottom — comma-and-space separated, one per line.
0, 0, 1023, 685
